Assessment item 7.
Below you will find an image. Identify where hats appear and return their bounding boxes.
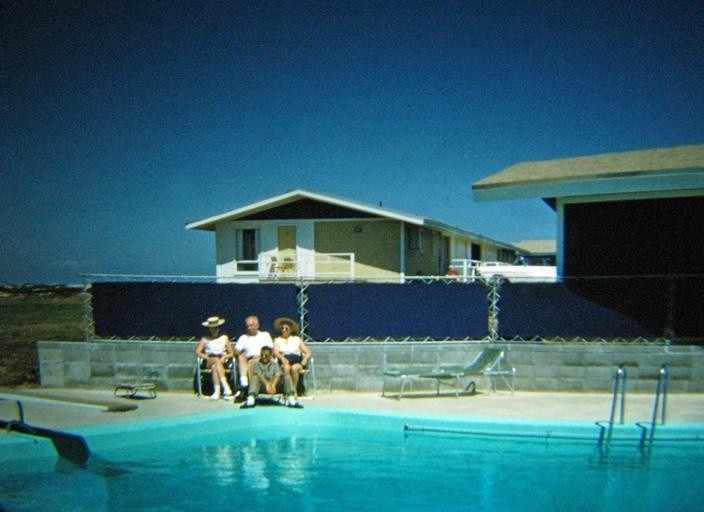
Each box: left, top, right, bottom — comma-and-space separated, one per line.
201, 316, 226, 328
274, 316, 300, 335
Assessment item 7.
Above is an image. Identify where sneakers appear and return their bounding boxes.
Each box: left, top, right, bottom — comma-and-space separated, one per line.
279, 395, 304, 407
235, 388, 247, 402
223, 390, 232, 399
209, 391, 220, 401
245, 396, 255, 408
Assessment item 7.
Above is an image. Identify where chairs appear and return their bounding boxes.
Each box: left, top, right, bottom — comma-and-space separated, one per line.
381, 347, 516, 400
114, 371, 158, 399
195, 355, 317, 406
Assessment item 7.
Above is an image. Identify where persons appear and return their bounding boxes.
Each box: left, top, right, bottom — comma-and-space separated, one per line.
234, 314, 274, 387
245, 346, 298, 407
195, 315, 233, 401
272, 316, 310, 400
